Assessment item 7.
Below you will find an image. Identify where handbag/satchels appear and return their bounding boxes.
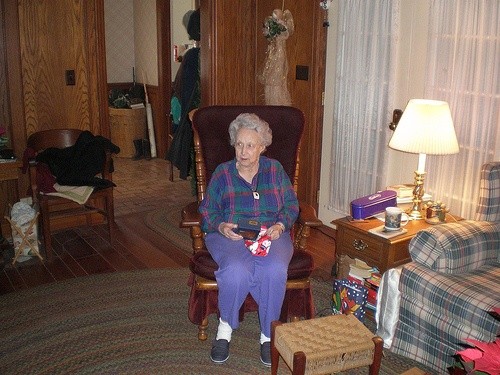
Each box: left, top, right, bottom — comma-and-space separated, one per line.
331, 277, 369, 323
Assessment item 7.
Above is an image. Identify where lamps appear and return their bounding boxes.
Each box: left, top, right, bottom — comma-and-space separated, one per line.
389, 99, 459, 221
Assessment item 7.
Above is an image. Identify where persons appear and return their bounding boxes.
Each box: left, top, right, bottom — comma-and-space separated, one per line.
198, 113, 300, 367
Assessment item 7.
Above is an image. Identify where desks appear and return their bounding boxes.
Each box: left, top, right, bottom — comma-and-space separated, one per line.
0, 161, 22, 263
330, 210, 463, 324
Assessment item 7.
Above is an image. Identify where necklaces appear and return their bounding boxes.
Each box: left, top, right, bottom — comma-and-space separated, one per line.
242, 173, 260, 200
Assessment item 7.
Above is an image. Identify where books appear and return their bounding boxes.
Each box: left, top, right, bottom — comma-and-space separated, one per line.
386, 184, 434, 212
346, 258, 382, 310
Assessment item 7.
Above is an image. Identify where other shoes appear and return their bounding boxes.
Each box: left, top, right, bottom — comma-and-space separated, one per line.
210, 334, 231, 362
259, 341, 271, 365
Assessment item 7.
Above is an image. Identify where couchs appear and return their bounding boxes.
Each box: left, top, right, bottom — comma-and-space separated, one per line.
374, 162, 500, 375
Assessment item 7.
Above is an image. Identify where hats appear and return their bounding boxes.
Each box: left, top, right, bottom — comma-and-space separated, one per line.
182, 10, 197, 41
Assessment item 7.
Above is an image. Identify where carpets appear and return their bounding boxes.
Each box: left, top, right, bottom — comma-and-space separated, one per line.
0, 267, 439, 375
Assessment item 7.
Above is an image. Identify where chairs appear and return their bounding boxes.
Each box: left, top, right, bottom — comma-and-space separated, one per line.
23, 129, 120, 254
179, 105, 323, 340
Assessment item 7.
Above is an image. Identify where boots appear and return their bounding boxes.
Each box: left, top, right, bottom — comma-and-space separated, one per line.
143, 140, 152, 160
131, 139, 144, 160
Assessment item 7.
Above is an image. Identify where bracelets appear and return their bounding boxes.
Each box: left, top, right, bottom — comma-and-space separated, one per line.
277, 223, 284, 234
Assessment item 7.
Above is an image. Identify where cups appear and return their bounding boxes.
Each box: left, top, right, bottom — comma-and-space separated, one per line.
385, 207, 409, 230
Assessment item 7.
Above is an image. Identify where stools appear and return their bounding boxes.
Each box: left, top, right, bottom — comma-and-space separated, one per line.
270, 313, 384, 375
